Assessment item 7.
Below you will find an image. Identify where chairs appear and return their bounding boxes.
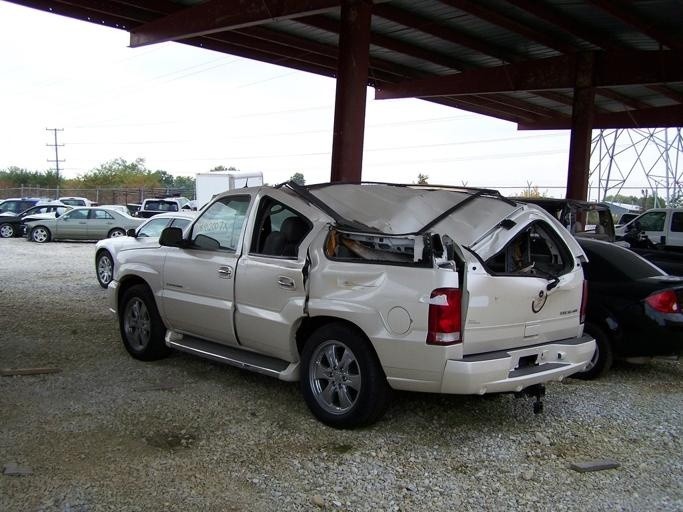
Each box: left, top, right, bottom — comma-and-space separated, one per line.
262, 216, 310, 257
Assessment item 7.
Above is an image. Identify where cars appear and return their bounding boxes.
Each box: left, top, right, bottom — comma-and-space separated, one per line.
106, 180, 596, 430
0, 193, 202, 290
503, 197, 683, 380
